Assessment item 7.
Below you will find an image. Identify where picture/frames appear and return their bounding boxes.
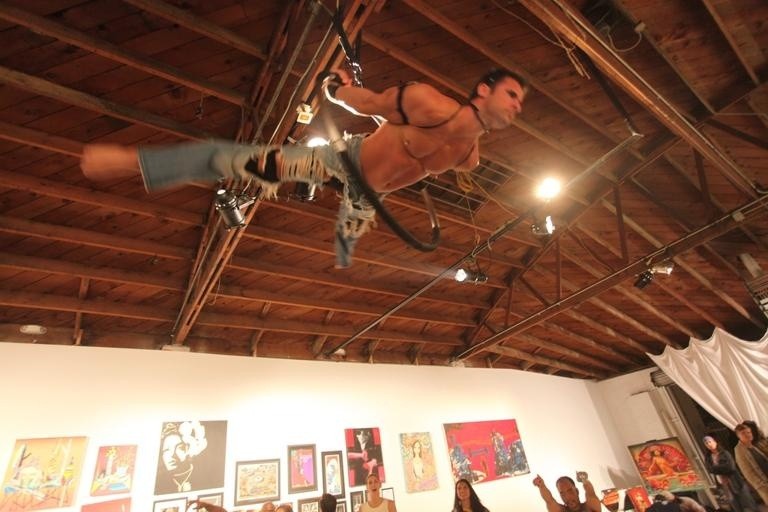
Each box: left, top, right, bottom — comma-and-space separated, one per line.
627, 486, 651, 512
90, 445, 137, 496
234, 459, 281, 507
0, 436, 89, 512
365, 489, 369, 501
322, 451, 345, 499
443, 418, 528, 486
197, 492, 224, 512
153, 497, 188, 512
288, 444, 318, 494
336, 501, 346, 512
80, 496, 131, 512
297, 496, 322, 512
382, 488, 395, 501
350, 491, 363, 512
154, 420, 227, 496
626, 436, 707, 497
400, 432, 439, 494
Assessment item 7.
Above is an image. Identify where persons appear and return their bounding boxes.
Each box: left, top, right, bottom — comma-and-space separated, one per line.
409, 439, 434, 481
276, 504, 293, 512
161, 507, 180, 512
450, 479, 490, 512
533, 471, 602, 512
319, 493, 337, 511
742, 420, 768, 444
261, 502, 275, 512
326, 458, 340, 492
635, 491, 647, 508
80, 67, 527, 271
644, 494, 680, 512
661, 491, 707, 512
703, 436, 758, 512
188, 499, 227, 512
648, 448, 683, 486
108, 453, 136, 492
161, 421, 208, 492
733, 425, 768, 506
93, 468, 117, 494
357, 474, 397, 512
447, 427, 531, 482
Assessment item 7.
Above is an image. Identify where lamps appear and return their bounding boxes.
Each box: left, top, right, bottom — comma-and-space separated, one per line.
216, 189, 245, 231
531, 216, 555, 236
633, 272, 653, 290
456, 268, 491, 285
649, 262, 673, 275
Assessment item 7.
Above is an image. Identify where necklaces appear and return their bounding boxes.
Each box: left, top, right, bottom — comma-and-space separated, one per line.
465, 99, 492, 140
